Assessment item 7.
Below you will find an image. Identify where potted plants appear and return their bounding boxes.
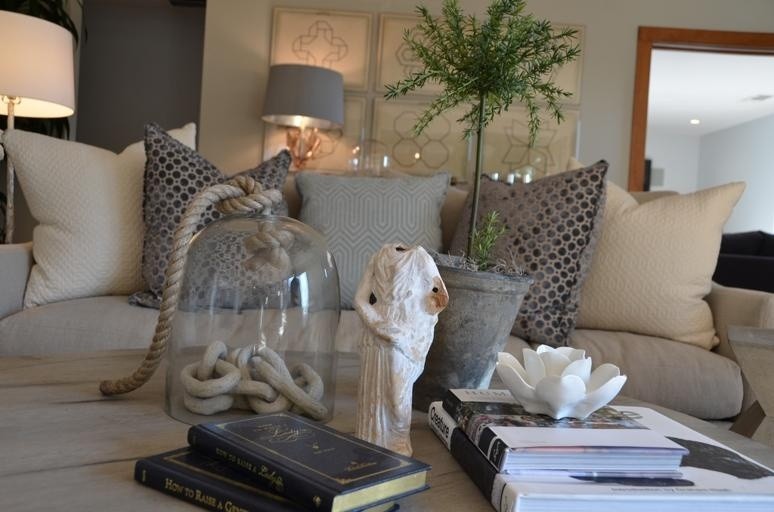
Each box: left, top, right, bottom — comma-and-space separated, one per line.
373, 4, 588, 415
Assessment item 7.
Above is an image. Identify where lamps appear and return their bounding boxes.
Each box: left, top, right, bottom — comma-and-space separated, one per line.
258, 62, 347, 172
0, 8, 78, 248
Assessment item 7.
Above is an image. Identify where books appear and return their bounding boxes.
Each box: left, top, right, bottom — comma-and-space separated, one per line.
429, 388, 774, 509
133, 409, 433, 512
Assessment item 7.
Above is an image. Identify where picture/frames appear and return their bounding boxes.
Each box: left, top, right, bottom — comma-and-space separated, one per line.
505, 26, 586, 104
379, 12, 478, 100
375, 102, 474, 179
270, 7, 370, 93
481, 104, 581, 179
267, 97, 365, 171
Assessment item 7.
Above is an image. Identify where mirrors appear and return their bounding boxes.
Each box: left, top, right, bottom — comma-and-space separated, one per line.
629, 24, 774, 292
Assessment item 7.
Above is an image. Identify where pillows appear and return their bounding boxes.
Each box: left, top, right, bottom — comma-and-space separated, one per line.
566, 178, 741, 351
446, 155, 610, 350
129, 118, 290, 311
279, 167, 450, 308
2, 119, 200, 317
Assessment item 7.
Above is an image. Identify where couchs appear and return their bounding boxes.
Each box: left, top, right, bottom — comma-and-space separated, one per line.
0, 210, 773, 472
